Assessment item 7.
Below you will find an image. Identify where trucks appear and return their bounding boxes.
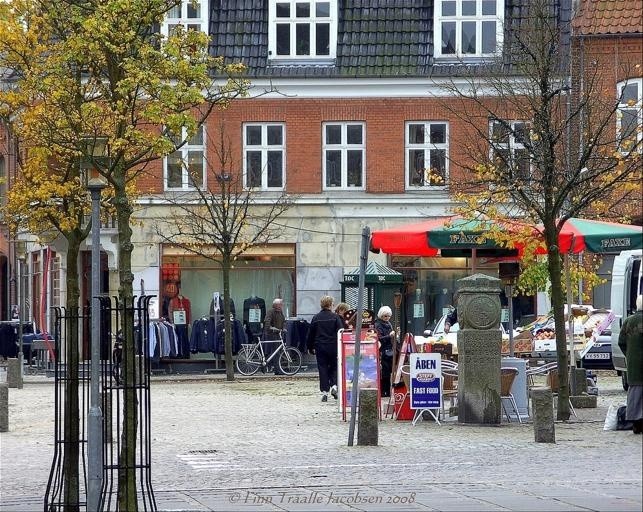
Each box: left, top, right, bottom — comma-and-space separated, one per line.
609, 249, 643, 389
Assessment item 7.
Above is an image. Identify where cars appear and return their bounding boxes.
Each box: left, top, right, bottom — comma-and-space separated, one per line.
576, 308, 612, 371
422, 313, 504, 353
0, 321, 53, 361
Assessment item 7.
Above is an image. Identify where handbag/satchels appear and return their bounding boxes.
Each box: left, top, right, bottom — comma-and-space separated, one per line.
603, 400, 633, 431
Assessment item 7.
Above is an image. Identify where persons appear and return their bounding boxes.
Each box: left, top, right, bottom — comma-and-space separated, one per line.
335, 303, 350, 327
307, 296, 344, 401
443, 290, 461, 333
262, 298, 286, 375
211, 294, 235, 319
243, 294, 265, 341
170, 294, 191, 327
618, 295, 642, 433
374, 305, 395, 396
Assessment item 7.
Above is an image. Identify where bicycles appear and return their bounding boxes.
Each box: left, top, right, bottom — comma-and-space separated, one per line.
236, 326, 303, 376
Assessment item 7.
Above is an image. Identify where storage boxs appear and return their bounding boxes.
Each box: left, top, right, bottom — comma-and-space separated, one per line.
417, 344, 423, 352
502, 339, 532, 353
423, 343, 432, 353
431, 344, 452, 355
535, 339, 557, 351
565, 334, 586, 350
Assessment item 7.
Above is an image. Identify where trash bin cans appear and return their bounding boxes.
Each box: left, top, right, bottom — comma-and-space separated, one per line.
392, 380, 416, 421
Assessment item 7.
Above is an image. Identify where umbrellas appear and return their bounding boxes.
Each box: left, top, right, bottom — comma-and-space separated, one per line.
528, 216, 643, 409
371, 207, 574, 274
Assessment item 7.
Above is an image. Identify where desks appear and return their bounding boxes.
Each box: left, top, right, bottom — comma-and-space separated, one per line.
16, 341, 55, 371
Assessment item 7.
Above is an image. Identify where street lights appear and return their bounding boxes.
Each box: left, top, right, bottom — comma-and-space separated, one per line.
77, 133, 111, 512
575, 167, 591, 304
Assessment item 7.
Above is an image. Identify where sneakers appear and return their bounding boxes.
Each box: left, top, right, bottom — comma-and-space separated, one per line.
331, 388, 337, 399
322, 395, 327, 401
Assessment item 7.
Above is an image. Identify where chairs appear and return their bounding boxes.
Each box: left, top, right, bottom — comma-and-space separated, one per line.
394, 360, 458, 423
500, 367, 522, 423
526, 362, 578, 418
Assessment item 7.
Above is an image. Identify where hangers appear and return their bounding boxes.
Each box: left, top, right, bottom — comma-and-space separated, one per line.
217, 314, 240, 325
193, 314, 213, 325
149, 317, 176, 330
286, 317, 311, 329
0, 321, 33, 332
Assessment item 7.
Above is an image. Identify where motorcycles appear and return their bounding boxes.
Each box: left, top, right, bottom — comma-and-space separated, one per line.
106, 329, 122, 386
